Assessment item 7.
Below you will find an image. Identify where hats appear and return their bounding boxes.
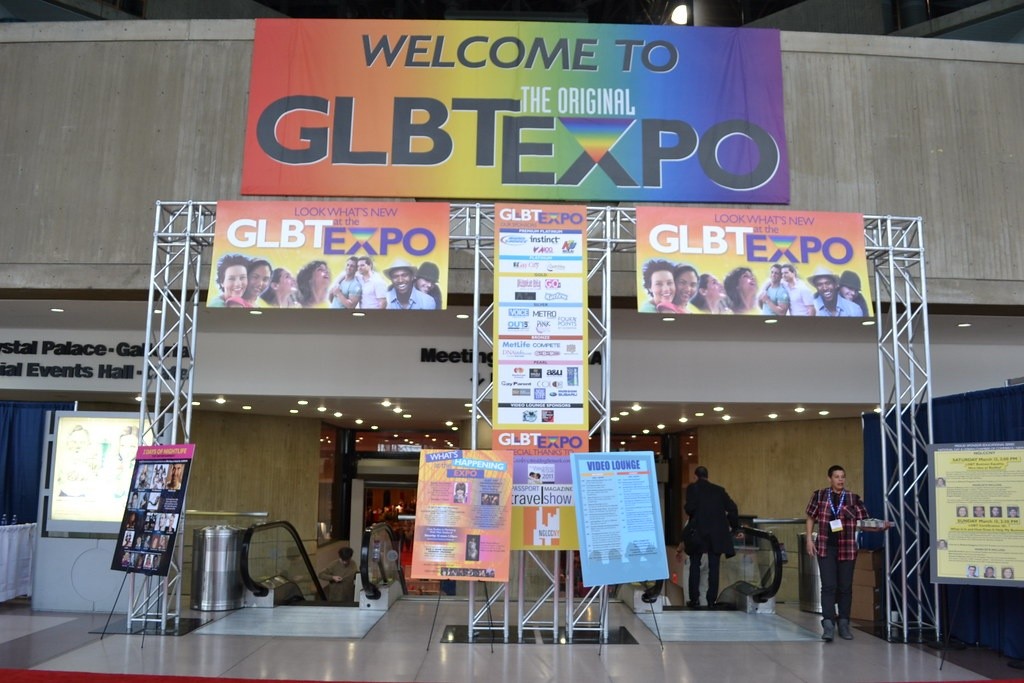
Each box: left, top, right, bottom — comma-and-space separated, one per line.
415, 262, 439, 283
807, 266, 839, 287
382, 259, 417, 280
839, 270, 863, 292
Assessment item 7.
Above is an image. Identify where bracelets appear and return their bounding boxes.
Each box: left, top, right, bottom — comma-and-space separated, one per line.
676, 549, 681, 553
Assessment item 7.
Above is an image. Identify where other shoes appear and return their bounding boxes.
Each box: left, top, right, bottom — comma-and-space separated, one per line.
686, 600, 700, 606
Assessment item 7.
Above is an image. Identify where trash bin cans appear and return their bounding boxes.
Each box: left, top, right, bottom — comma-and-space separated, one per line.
190, 525, 248, 612
798, 531, 838, 614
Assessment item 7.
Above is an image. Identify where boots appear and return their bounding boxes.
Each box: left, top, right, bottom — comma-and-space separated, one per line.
822, 620, 834, 641
837, 618, 854, 639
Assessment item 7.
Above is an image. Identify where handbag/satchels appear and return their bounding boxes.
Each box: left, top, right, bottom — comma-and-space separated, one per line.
682, 518, 701, 556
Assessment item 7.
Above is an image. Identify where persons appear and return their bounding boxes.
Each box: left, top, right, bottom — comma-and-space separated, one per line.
526, 472, 543, 486
119, 461, 185, 571
936, 478, 1020, 579
638, 257, 871, 317
675, 510, 710, 606
205, 252, 445, 310
318, 546, 358, 603
680, 465, 739, 608
805, 465, 891, 642
454, 483, 467, 504
